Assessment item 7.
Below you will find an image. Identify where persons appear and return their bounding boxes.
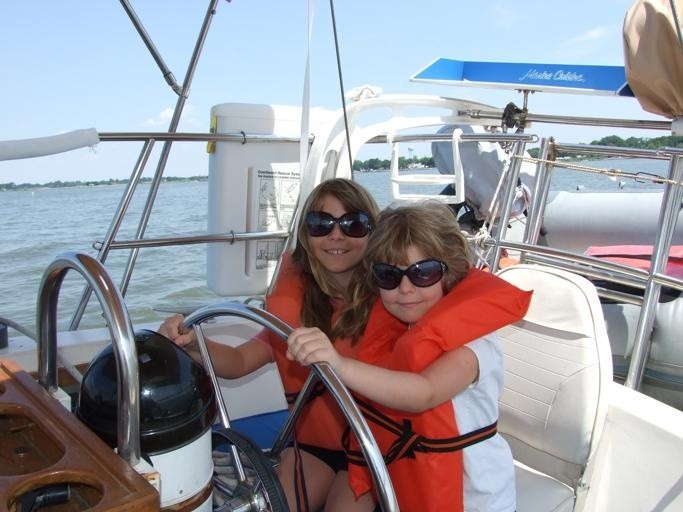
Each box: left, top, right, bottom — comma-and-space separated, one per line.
285, 199, 517, 512
157, 179, 379, 512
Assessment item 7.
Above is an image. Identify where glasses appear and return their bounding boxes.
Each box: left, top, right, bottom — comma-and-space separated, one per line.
305, 209, 373, 237
367, 260, 448, 289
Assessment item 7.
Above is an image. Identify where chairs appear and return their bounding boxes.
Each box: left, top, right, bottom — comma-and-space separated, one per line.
481, 263, 614, 509
